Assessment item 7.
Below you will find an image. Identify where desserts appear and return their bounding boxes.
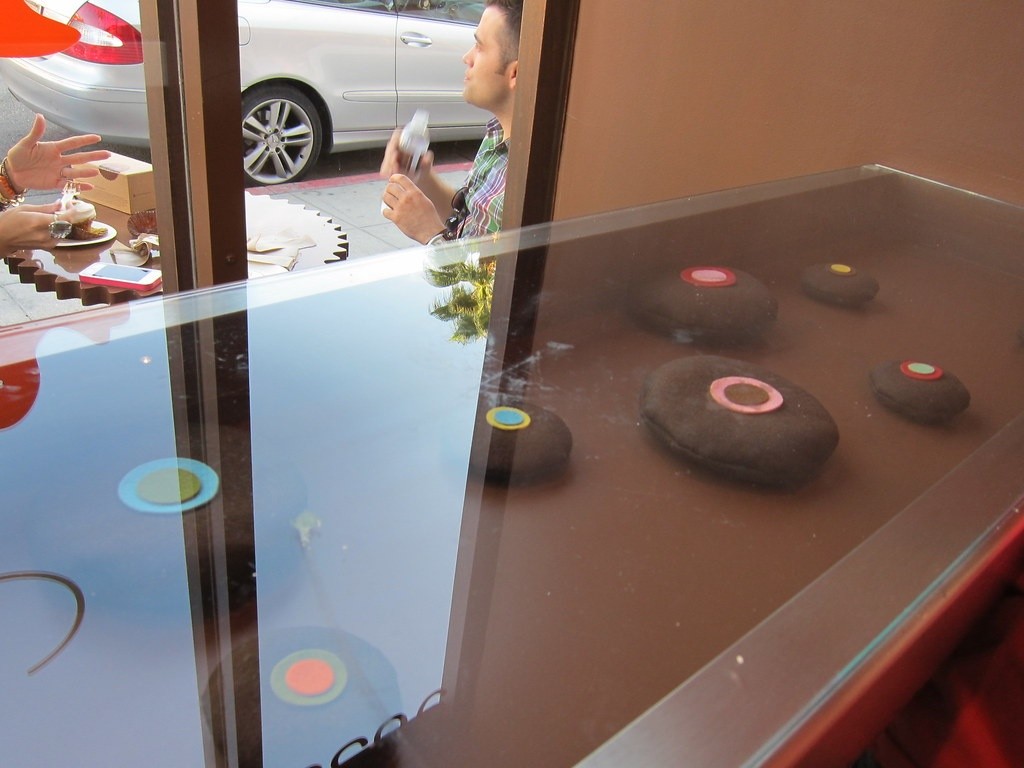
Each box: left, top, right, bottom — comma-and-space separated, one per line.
52, 199, 96, 232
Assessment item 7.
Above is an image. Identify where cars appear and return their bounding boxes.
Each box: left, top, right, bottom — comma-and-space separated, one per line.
1, 1, 499, 189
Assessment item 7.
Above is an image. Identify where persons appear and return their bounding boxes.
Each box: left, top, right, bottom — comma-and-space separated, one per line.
378, 0, 525, 248
0, 114, 111, 260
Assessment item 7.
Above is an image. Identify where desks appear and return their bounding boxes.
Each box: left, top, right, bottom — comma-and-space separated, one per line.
4, 187, 349, 304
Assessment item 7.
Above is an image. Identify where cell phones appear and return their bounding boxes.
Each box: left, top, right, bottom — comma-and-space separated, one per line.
79, 262, 162, 291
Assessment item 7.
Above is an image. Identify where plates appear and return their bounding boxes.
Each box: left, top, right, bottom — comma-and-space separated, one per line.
52, 215, 117, 246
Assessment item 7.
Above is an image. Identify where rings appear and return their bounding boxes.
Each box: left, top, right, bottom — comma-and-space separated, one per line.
61, 172, 62, 175
49, 221, 72, 239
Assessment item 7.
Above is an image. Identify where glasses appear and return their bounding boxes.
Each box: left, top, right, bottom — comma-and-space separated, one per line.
442, 185, 470, 241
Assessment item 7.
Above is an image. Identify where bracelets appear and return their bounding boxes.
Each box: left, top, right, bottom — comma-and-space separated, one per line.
427, 234, 443, 245
0, 158, 28, 210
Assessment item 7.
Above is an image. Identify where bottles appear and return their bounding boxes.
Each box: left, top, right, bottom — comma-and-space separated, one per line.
380, 109, 431, 219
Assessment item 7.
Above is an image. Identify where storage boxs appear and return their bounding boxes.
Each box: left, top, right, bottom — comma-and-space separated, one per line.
70, 150, 155, 216
